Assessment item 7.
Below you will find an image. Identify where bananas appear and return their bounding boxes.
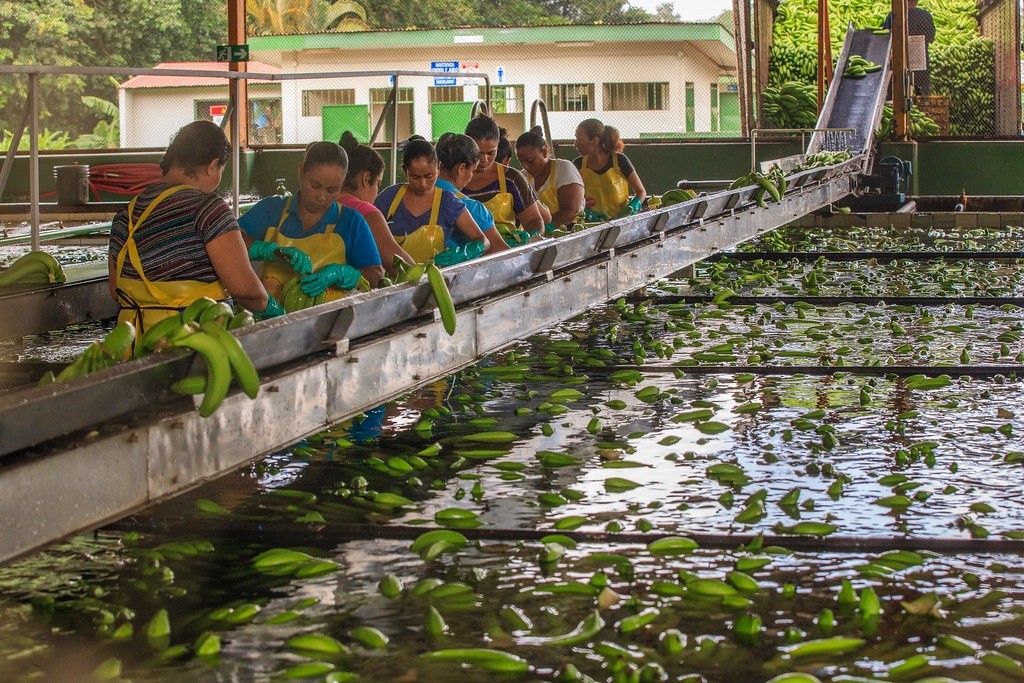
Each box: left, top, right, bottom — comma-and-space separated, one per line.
1, 0, 1023, 682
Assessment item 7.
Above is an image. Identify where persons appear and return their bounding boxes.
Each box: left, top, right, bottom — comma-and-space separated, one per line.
883, 0, 935, 101
236, 118, 647, 319
108, 121, 287, 359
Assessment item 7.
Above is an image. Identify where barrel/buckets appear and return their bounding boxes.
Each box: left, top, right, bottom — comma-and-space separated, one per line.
54, 165, 90, 206
